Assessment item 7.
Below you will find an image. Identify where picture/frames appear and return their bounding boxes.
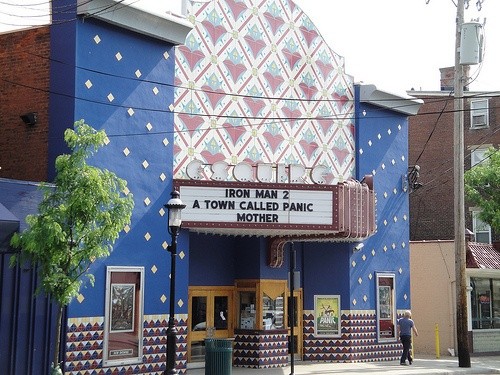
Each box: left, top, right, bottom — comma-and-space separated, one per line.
313, 293, 342, 339
110, 283, 136, 334
379, 285, 392, 321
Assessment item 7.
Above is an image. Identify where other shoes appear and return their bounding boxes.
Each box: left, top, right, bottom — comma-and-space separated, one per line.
409, 359, 413, 364
400, 362, 406, 365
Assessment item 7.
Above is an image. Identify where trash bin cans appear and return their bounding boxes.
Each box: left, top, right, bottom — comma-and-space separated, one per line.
203, 337, 235, 375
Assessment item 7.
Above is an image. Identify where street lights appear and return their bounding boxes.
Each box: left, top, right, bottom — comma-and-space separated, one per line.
161, 186, 185, 375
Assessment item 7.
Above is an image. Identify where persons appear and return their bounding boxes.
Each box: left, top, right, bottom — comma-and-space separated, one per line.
397, 311, 418, 365
